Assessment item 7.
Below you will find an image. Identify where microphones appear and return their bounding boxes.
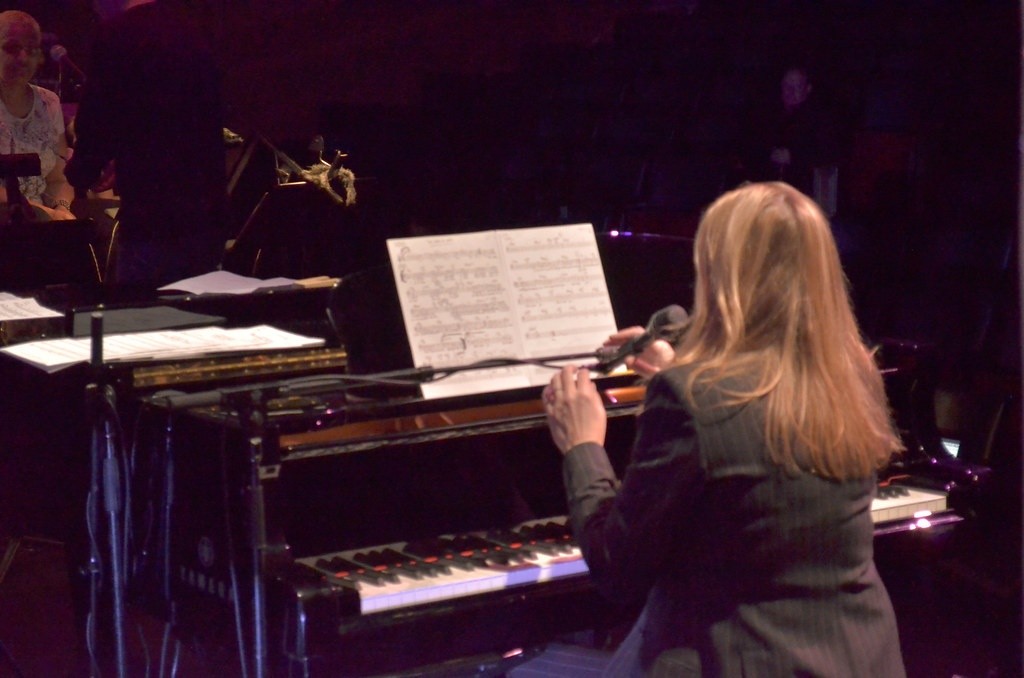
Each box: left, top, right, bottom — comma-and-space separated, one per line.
596, 305, 688, 375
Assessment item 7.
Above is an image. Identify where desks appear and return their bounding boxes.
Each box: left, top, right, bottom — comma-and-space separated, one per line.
25, 319, 347, 587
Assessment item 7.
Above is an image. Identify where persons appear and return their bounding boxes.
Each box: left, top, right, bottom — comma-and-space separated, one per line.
541, 178, 911, 678
729, 60, 849, 192
0, 1, 235, 328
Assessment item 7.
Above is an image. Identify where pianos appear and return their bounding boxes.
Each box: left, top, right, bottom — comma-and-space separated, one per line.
120, 334, 994, 678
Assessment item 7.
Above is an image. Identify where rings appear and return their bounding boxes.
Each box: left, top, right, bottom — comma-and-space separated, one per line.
552, 387, 563, 393
546, 390, 554, 402
542, 399, 549, 408
